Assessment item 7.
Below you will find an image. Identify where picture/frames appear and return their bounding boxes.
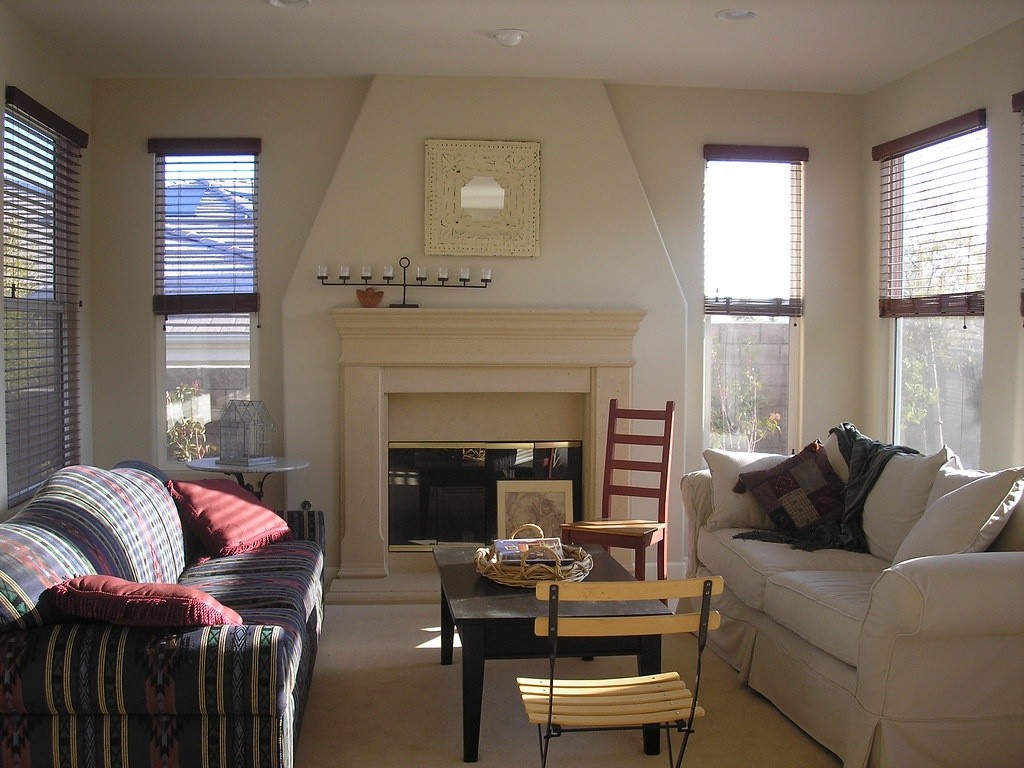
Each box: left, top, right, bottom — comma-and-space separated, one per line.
496, 480, 573, 540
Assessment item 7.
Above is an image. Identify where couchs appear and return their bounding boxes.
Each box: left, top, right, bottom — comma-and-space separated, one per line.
680, 462, 1024, 768
0, 460, 326, 768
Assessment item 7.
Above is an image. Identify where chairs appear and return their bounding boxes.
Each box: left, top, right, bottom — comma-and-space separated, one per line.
561, 399, 676, 605
516, 575, 724, 768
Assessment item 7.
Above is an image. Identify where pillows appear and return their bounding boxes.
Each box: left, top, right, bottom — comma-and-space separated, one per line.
45, 574, 243, 626
701, 421, 1024, 567
165, 478, 293, 556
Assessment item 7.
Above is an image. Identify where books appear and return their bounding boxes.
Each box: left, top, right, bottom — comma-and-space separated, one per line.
493, 538, 574, 566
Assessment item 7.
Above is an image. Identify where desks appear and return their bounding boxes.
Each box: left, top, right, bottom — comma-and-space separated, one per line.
186, 457, 309, 502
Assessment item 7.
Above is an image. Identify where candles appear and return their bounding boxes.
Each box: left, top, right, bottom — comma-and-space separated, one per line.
317, 266, 394, 285
416, 266, 492, 288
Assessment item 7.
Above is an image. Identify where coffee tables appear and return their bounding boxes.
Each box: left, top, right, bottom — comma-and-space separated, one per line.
431, 543, 674, 763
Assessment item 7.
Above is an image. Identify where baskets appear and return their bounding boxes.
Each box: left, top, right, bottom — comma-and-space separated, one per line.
475, 522, 594, 588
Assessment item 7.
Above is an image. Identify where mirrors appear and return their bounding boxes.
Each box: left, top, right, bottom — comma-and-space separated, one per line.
461, 175, 505, 218
